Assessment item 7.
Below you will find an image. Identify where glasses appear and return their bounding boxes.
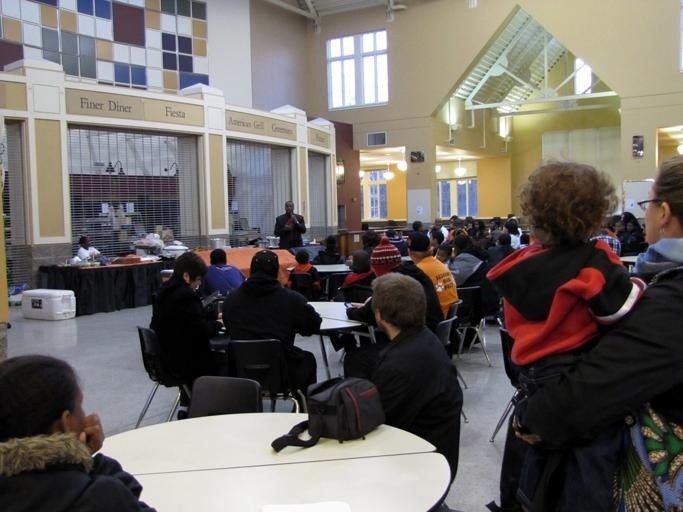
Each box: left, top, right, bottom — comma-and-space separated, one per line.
635, 197, 664, 212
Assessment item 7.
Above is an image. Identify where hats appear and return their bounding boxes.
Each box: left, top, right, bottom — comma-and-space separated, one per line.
405, 231, 430, 252
360, 229, 382, 249
250, 250, 280, 275
367, 236, 402, 277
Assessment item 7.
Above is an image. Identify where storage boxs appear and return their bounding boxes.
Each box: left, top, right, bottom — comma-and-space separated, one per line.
22, 288, 76, 320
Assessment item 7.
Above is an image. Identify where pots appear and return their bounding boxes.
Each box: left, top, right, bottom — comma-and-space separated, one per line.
210, 239, 224, 248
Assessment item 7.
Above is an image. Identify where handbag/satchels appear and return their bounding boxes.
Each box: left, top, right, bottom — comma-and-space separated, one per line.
269, 372, 387, 452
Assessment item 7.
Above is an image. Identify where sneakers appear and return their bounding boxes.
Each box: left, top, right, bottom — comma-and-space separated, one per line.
176, 409, 188, 421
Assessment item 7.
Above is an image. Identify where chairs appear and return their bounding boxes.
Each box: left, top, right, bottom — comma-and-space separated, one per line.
490, 328, 527, 442
187, 376, 264, 419
436, 285, 499, 422
226, 338, 282, 412
134, 325, 192, 429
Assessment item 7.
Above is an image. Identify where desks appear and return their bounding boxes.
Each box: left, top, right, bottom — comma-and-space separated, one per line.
286, 263, 355, 301
39, 260, 177, 317
306, 300, 377, 381
91, 412, 453, 512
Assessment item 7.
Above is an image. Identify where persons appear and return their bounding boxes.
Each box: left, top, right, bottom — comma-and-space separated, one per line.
313, 236, 341, 266
506, 155, 682, 509
348, 272, 463, 512
77, 236, 111, 266
199, 249, 247, 298
348, 215, 529, 353
589, 210, 649, 259
288, 251, 319, 297
221, 250, 321, 412
148, 253, 224, 419
275, 200, 304, 248
484, 160, 650, 508
0, 356, 155, 511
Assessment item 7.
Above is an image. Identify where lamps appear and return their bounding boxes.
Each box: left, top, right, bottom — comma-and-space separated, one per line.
454, 157, 466, 176
383, 162, 394, 180
397, 149, 407, 171
